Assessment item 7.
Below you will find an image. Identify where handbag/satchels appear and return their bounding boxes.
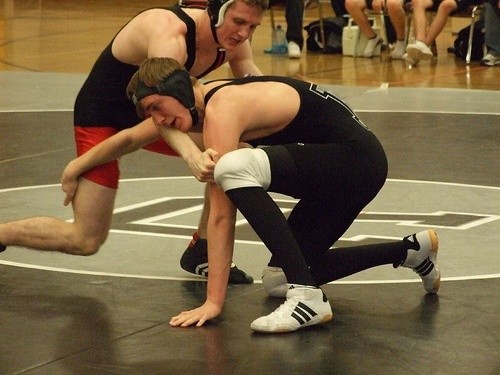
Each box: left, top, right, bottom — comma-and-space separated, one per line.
307, 17, 347, 53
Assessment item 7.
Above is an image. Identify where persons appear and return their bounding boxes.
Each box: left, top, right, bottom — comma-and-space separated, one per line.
0, 0, 268, 255
62, 57, 439, 334
285, 0, 500, 67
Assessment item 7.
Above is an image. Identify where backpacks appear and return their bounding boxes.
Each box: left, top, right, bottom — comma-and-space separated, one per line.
454, 19, 486, 61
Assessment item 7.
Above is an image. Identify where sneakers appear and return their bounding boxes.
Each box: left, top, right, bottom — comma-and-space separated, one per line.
480, 53, 500, 66
403, 41, 433, 66
392, 40, 407, 59
364, 36, 383, 57
250, 287, 333, 333
399, 228, 440, 295
180, 232, 254, 284
287, 41, 300, 58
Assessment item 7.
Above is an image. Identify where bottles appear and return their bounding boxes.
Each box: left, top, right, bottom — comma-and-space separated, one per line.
272, 25, 288, 54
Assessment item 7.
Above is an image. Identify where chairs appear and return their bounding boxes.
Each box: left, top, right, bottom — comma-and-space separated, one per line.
270, 0, 487, 62
